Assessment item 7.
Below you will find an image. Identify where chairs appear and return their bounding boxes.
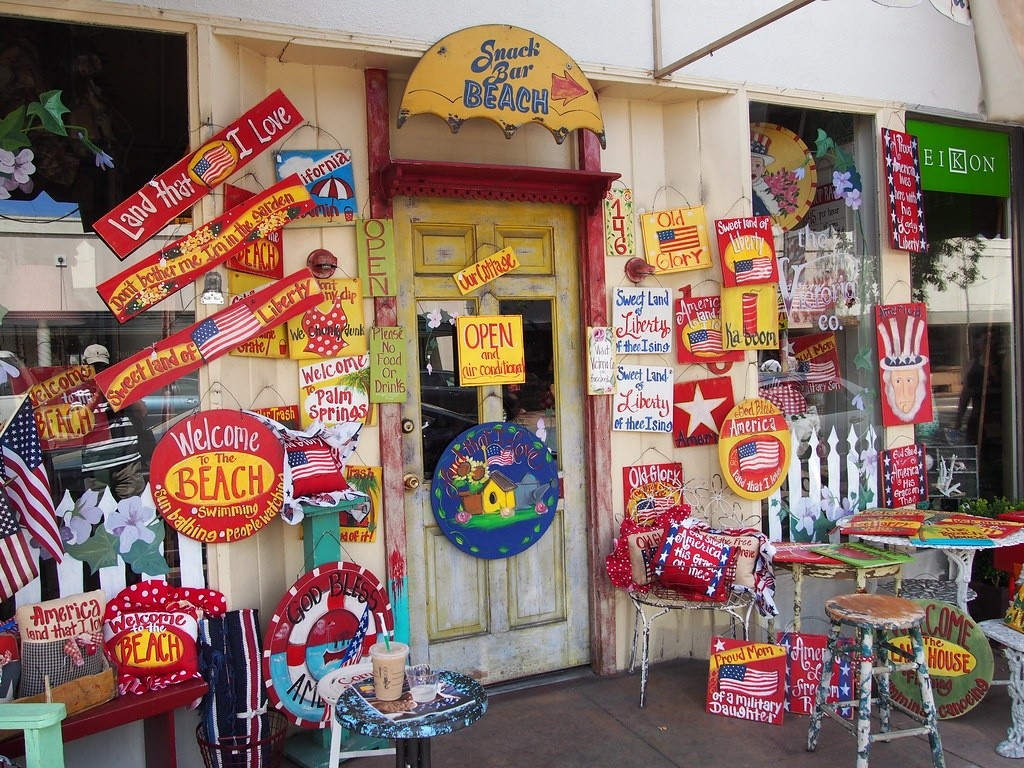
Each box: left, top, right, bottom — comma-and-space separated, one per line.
976, 559, 1024, 760
626, 471, 755, 711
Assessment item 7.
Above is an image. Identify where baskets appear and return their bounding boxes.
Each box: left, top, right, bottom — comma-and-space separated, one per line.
195, 706, 288, 767
646, 552, 736, 601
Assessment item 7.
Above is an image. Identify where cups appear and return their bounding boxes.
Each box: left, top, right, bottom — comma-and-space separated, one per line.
370, 641, 410, 701
405, 663, 439, 703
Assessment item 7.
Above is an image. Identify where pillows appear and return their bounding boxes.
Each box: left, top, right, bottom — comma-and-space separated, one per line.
604, 527, 666, 593
724, 528, 759, 591
648, 520, 740, 605
17, 588, 107, 698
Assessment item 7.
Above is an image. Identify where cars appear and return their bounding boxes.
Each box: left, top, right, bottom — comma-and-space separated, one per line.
419, 365, 481, 477
758, 369, 985, 504
0, 347, 201, 499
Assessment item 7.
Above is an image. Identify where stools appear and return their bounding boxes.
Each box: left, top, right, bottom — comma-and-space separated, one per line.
806, 593, 947, 768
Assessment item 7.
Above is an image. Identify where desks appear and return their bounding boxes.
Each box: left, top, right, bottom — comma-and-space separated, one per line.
835, 505, 1024, 612
332, 666, 488, 768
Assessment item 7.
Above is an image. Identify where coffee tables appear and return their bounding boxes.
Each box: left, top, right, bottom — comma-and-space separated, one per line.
315, 662, 411, 768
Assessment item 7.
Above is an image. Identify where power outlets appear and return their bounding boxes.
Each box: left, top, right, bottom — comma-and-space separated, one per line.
56, 255, 67, 267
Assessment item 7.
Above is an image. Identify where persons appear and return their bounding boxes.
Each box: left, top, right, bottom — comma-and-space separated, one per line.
70, 343, 153, 501
954, 327, 1007, 501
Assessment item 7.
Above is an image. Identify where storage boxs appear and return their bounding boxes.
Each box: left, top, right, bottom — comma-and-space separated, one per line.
194, 706, 289, 768
647, 557, 738, 604
